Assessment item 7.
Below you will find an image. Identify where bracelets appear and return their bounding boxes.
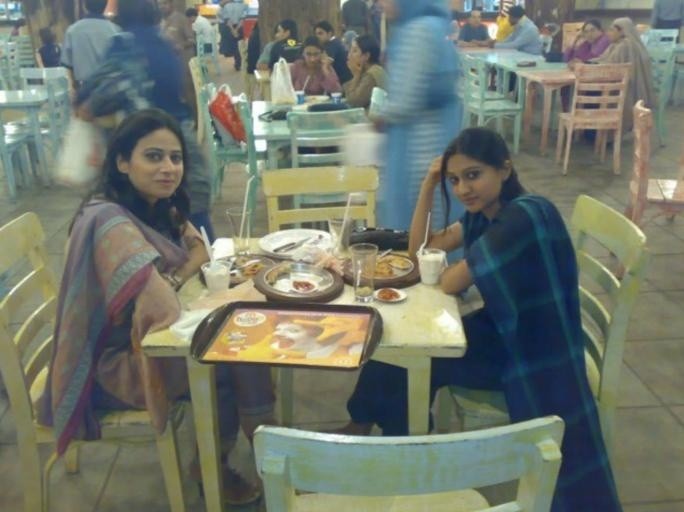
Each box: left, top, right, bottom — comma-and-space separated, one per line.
167, 273, 184, 289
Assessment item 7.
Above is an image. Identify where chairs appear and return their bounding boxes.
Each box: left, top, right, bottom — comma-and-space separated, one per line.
0, 121, 34, 198
431, 194, 648, 463
263, 165, 380, 236
286, 107, 366, 227
614, 100, 684, 280
642, 29, 680, 147
0, 211, 186, 511
556, 61, 634, 176
239, 103, 292, 235
199, 91, 248, 199
461, 57, 524, 158
25, 78, 70, 177
196, 34, 212, 84
252, 415, 566, 512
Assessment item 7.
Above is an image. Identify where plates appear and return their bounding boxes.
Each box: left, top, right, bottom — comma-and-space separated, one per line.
190, 300, 383, 371
200, 255, 277, 288
374, 287, 407, 302
253, 260, 344, 301
343, 252, 421, 288
259, 228, 333, 259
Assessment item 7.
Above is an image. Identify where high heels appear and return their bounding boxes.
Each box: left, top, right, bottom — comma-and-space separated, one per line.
198, 465, 260, 504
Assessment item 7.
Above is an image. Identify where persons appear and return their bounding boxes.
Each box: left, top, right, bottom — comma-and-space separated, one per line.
346, 35, 391, 106
487, 5, 543, 92
218, 18, 241, 72
289, 37, 344, 95
458, 10, 491, 48
36, 109, 278, 506
496, 0, 516, 42
569, 17, 656, 143
74, 0, 216, 252
269, 19, 304, 74
257, 22, 278, 70
35, 27, 61, 68
369, 0, 467, 300
184, 8, 221, 80
316, 127, 623, 512
158, 1, 195, 105
61, 0, 124, 108
9, 18, 27, 43
366, 0, 384, 61
561, 20, 611, 113
342, 0, 368, 39
314, 20, 353, 85
223, 0, 249, 38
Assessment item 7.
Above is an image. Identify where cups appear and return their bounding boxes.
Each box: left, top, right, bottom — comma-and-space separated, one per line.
349, 243, 378, 302
226, 206, 252, 256
332, 92, 342, 104
296, 91, 305, 104
417, 248, 446, 284
328, 218, 350, 261
201, 262, 231, 296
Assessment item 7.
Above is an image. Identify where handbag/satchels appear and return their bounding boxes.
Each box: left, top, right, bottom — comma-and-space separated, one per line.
209, 85, 251, 149
349, 230, 409, 250
